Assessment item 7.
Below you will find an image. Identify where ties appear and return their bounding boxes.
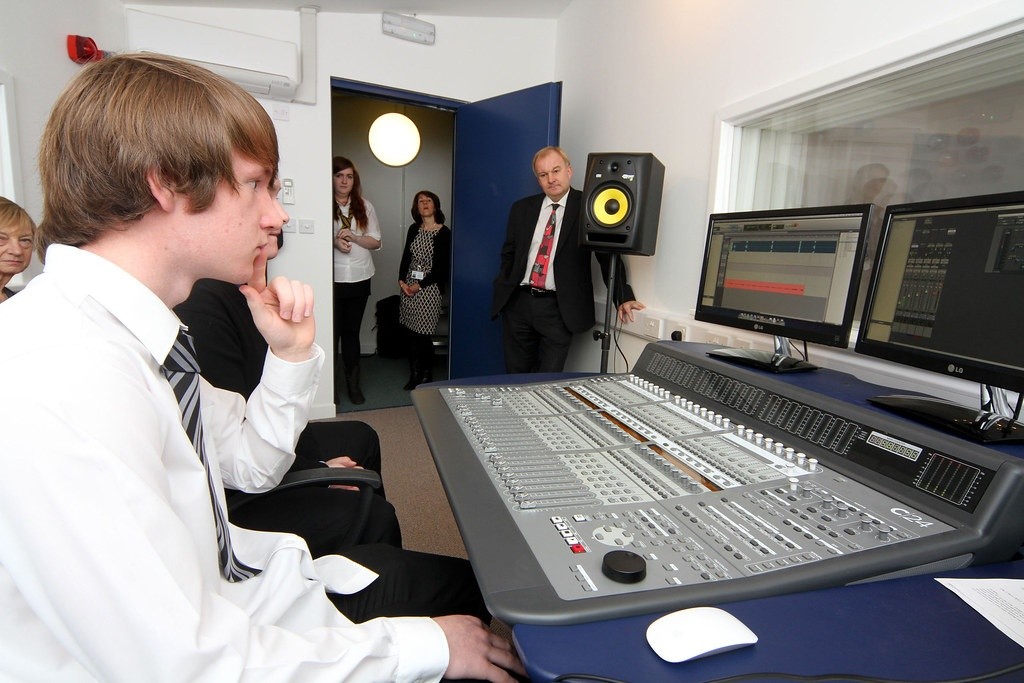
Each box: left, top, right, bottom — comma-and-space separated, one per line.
529, 204, 560, 290
162, 329, 262, 583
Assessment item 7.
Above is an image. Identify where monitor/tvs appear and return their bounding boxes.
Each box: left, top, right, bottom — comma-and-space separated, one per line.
853, 191, 1024, 444
695, 203, 875, 375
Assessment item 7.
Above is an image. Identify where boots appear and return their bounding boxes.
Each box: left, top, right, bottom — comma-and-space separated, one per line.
344, 362, 365, 405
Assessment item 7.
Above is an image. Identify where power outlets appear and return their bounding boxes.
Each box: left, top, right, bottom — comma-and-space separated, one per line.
675, 324, 689, 341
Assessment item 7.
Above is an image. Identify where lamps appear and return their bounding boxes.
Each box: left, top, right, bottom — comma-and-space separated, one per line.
379, 9, 438, 48
366, 102, 423, 167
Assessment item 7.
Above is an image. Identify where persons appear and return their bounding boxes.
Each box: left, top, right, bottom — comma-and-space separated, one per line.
494, 145, 645, 373
0, 53, 532, 683
397, 190, 452, 390
332, 156, 383, 404
173, 177, 492, 626
0, 196, 37, 304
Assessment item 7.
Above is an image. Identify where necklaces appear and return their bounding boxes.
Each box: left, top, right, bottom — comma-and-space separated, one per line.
336, 195, 349, 206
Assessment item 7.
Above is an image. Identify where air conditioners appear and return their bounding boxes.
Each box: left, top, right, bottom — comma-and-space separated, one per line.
121, 7, 302, 104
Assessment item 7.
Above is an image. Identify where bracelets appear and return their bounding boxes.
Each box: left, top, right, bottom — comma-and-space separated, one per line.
400, 282, 404, 287
418, 284, 421, 290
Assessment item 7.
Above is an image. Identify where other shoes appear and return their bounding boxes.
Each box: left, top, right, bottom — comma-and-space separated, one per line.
404, 377, 419, 390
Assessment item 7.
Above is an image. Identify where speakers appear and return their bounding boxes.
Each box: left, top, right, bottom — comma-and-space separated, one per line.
578, 152, 665, 257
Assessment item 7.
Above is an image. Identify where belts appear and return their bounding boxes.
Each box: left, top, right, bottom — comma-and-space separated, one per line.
520, 285, 555, 298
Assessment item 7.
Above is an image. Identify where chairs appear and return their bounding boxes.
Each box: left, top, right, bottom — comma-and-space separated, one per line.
218, 466, 383, 558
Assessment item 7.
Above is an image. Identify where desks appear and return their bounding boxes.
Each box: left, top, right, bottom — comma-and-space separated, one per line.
408, 337, 1024, 683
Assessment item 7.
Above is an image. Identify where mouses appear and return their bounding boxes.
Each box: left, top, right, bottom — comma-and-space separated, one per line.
645, 606, 759, 662
